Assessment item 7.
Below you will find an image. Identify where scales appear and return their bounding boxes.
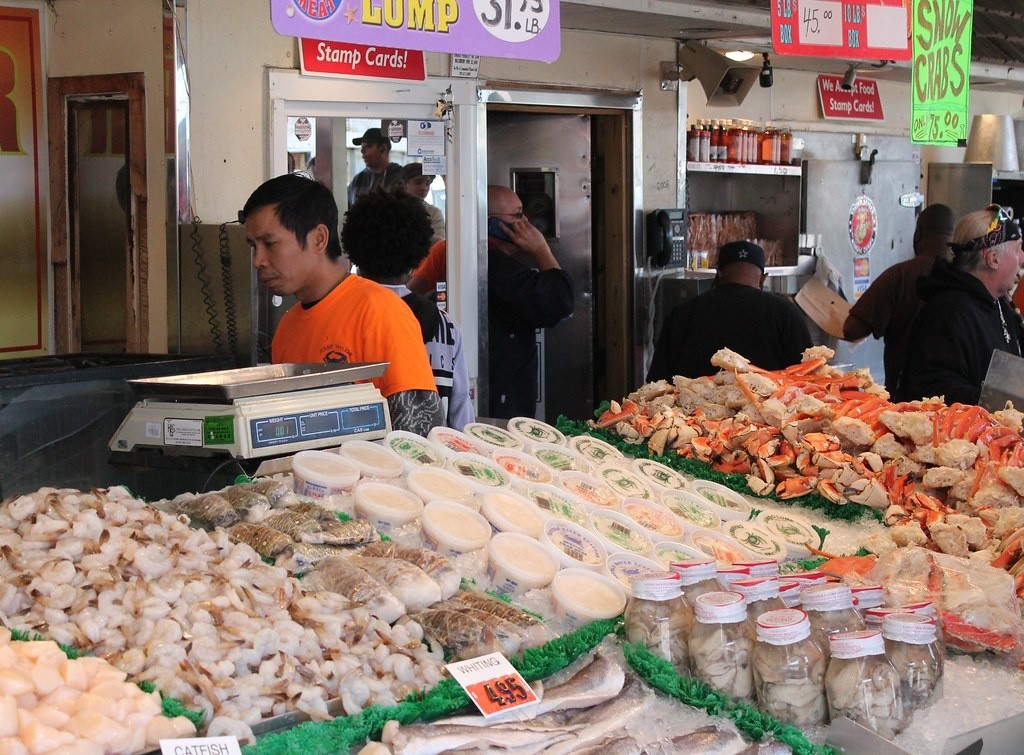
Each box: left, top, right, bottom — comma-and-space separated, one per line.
106, 362, 393, 460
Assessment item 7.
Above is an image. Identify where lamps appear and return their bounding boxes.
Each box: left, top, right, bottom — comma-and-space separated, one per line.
841, 62, 861, 90
759, 52, 773, 87
658, 40, 762, 107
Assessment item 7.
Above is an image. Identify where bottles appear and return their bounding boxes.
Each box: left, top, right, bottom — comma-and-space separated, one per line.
687, 117, 793, 165
624, 558, 947, 739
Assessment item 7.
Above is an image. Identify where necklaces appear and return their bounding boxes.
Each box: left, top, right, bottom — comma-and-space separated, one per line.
996, 298, 1011, 344
892, 200, 1024, 411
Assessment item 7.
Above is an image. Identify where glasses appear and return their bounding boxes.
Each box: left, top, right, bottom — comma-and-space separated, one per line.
488, 209, 523, 219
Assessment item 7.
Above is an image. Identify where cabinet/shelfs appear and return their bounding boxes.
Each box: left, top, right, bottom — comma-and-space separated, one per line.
684, 159, 805, 280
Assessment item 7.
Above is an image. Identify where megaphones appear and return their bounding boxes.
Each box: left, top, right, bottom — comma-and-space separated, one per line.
678, 39, 762, 108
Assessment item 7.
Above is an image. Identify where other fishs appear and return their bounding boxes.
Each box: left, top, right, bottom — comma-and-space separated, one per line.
355, 650, 793, 755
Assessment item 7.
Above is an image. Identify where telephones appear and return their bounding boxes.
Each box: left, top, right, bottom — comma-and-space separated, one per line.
646, 208, 689, 270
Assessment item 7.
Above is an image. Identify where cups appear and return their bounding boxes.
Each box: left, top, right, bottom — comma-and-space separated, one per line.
291, 416, 821, 626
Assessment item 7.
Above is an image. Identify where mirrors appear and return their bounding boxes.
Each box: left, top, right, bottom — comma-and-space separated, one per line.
261, 101, 474, 421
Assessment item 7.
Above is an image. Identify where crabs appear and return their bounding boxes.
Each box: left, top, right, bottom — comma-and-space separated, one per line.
585, 344, 1024, 671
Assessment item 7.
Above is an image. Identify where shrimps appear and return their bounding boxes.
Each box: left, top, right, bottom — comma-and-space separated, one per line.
0, 476, 554, 755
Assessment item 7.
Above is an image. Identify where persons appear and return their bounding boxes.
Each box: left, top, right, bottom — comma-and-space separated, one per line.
842, 203, 958, 406
400, 162, 446, 247
645, 238, 811, 385
486, 183, 575, 419
404, 238, 447, 296
346, 127, 406, 208
237, 174, 444, 437
340, 183, 478, 432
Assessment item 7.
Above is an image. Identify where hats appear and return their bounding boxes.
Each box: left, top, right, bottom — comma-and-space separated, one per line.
710, 242, 764, 288
352, 128, 392, 150
401, 164, 436, 187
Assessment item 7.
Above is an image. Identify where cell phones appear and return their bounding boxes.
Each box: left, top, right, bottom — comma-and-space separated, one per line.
488, 217, 515, 243
